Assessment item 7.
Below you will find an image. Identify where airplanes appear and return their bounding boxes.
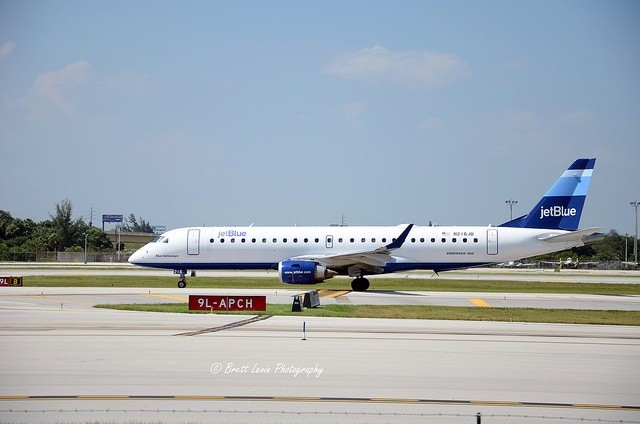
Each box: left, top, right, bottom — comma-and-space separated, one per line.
128, 158, 603, 290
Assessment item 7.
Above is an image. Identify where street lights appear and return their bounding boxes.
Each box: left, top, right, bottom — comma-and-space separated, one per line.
630, 202, 640, 263
506, 201, 518, 221
